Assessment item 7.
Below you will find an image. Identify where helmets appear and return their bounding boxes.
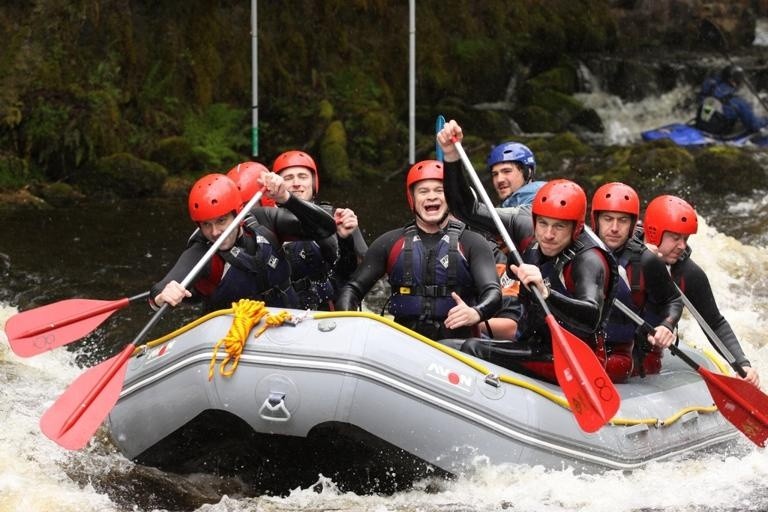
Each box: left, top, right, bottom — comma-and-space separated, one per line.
407, 160, 443, 213
488, 142, 535, 183
722, 65, 745, 91
532, 179, 587, 242
644, 195, 698, 246
591, 183, 640, 238
272, 151, 318, 199
225, 162, 275, 207
189, 174, 244, 223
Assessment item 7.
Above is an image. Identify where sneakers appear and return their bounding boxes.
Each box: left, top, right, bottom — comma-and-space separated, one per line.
761, 126, 768, 138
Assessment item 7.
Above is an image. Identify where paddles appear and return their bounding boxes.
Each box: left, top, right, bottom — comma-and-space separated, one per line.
41, 173, 281, 450
613, 297, 768, 448
450, 130, 621, 433
4, 288, 151, 357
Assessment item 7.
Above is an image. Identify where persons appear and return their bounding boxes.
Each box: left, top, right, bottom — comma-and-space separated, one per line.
478, 239, 521, 342
338, 160, 503, 341
591, 183, 684, 384
634, 195, 761, 390
226, 161, 338, 311
436, 121, 619, 387
273, 152, 368, 265
486, 142, 548, 207
148, 174, 338, 319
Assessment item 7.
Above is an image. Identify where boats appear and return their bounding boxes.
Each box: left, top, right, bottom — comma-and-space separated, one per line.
641, 120, 768, 150
63, 299, 749, 479
